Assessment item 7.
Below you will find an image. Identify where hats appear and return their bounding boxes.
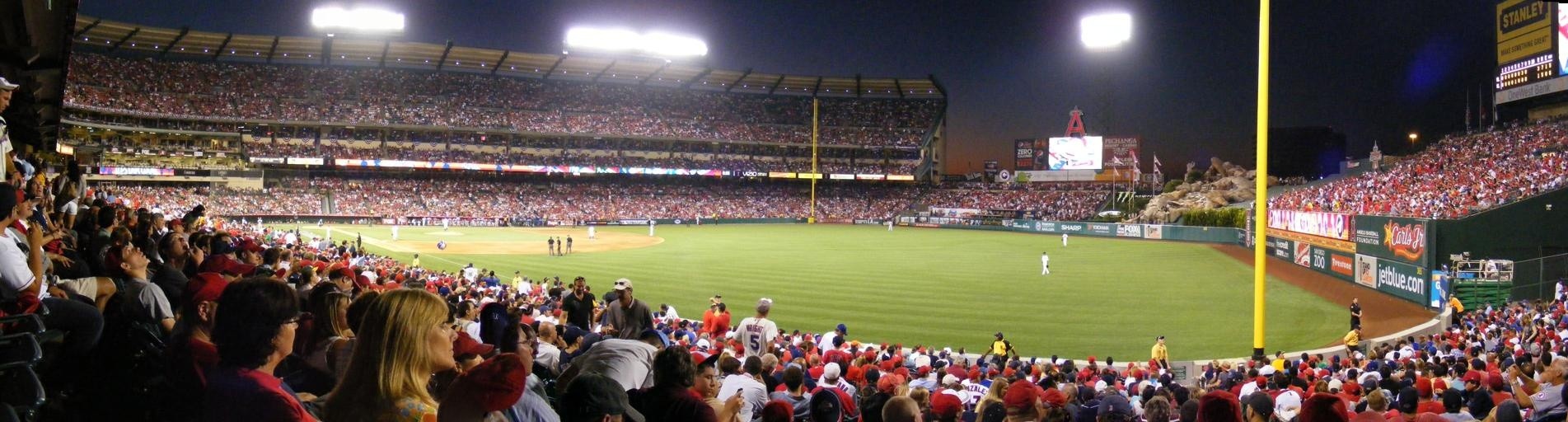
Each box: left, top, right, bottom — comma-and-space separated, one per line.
16, 188, 35, 203
0, 76, 22, 92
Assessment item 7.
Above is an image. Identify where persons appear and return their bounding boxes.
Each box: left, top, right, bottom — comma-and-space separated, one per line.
71, 52, 924, 223
0, 76, 1568, 422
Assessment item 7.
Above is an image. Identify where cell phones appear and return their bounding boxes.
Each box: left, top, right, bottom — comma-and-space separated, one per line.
736, 388, 742, 397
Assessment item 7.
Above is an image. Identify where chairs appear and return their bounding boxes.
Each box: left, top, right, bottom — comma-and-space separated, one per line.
0, 285, 175, 422
